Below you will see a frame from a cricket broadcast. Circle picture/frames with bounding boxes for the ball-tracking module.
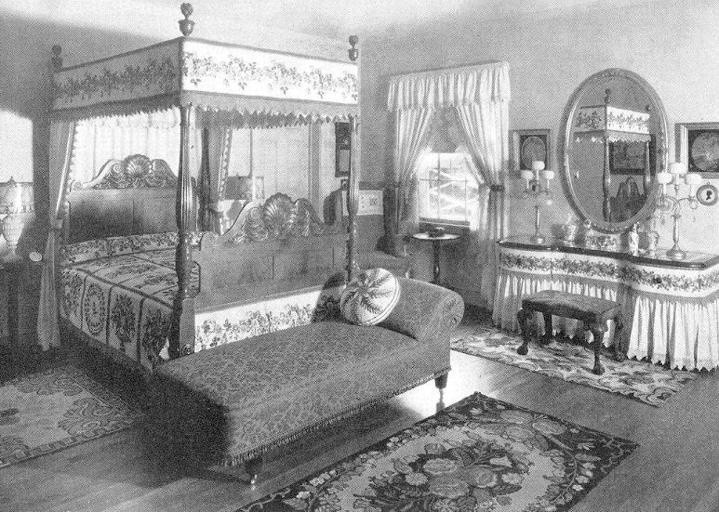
[607,133,655,178]
[677,121,719,180]
[510,127,553,175]
[696,182,719,207]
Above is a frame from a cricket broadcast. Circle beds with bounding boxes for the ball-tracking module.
[40,154,359,376]
[150,274,462,484]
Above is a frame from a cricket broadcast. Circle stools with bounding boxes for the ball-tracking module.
[518,282,624,373]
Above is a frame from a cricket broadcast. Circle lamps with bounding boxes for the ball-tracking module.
[659,163,707,261]
[521,159,555,244]
[0,177,35,264]
[228,174,265,202]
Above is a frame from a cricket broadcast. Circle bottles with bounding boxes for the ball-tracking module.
[560,218,660,256]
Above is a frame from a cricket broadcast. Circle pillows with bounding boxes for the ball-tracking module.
[338,266,403,325]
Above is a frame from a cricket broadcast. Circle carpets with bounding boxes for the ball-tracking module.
[1,361,149,468]
[230,389,641,511]
[450,324,697,405]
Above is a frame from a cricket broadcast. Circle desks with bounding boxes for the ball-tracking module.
[497,232,719,352]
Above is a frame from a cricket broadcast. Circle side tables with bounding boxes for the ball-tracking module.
[411,231,465,291]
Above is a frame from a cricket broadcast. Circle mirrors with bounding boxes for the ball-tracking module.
[556,66,670,236]
